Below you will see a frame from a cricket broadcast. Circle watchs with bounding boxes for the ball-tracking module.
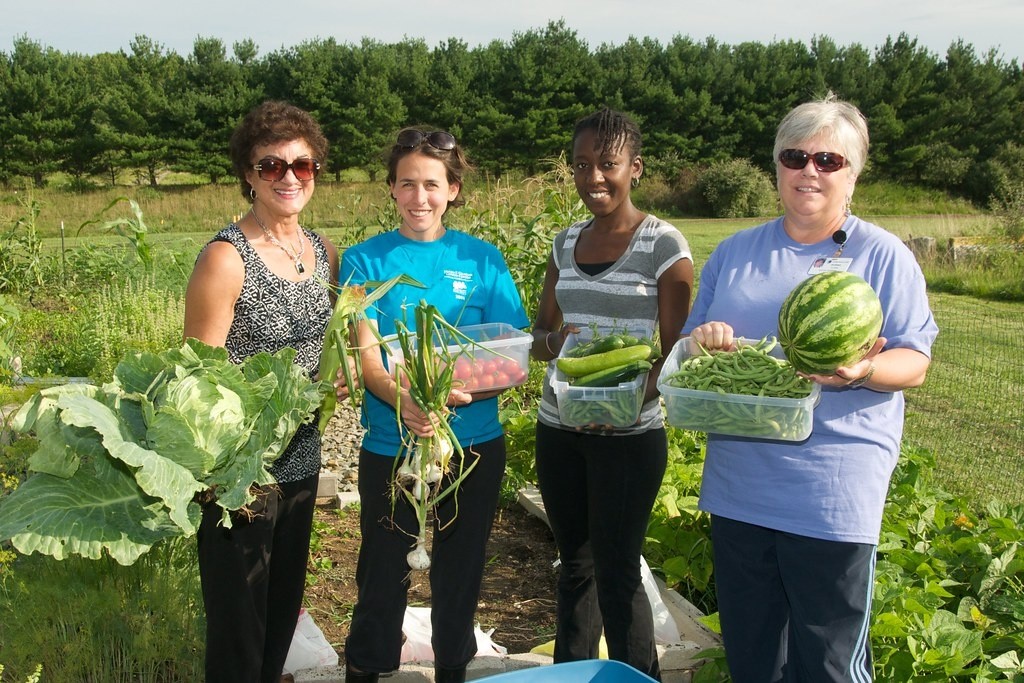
[848,366,875,389]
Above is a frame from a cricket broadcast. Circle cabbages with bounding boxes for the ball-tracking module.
[2,337,335,566]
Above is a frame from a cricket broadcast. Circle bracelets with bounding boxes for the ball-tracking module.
[546,331,561,357]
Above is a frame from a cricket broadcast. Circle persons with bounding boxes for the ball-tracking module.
[338,124,531,683]
[813,258,826,267]
[529,108,695,683]
[680,91,938,683]
[183,104,359,683]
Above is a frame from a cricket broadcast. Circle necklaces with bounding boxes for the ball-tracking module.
[250,206,305,275]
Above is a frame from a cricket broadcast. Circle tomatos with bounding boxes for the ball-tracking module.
[392,358,526,391]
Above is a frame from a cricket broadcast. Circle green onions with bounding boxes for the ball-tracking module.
[350,287,519,584]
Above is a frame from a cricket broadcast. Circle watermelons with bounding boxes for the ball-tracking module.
[779,271,884,376]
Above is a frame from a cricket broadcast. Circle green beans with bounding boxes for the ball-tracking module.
[659,333,814,440]
[567,319,630,353]
[560,388,642,426]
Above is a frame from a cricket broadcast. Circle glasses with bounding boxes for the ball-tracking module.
[778,148,852,172]
[247,156,321,182]
[395,128,457,150]
